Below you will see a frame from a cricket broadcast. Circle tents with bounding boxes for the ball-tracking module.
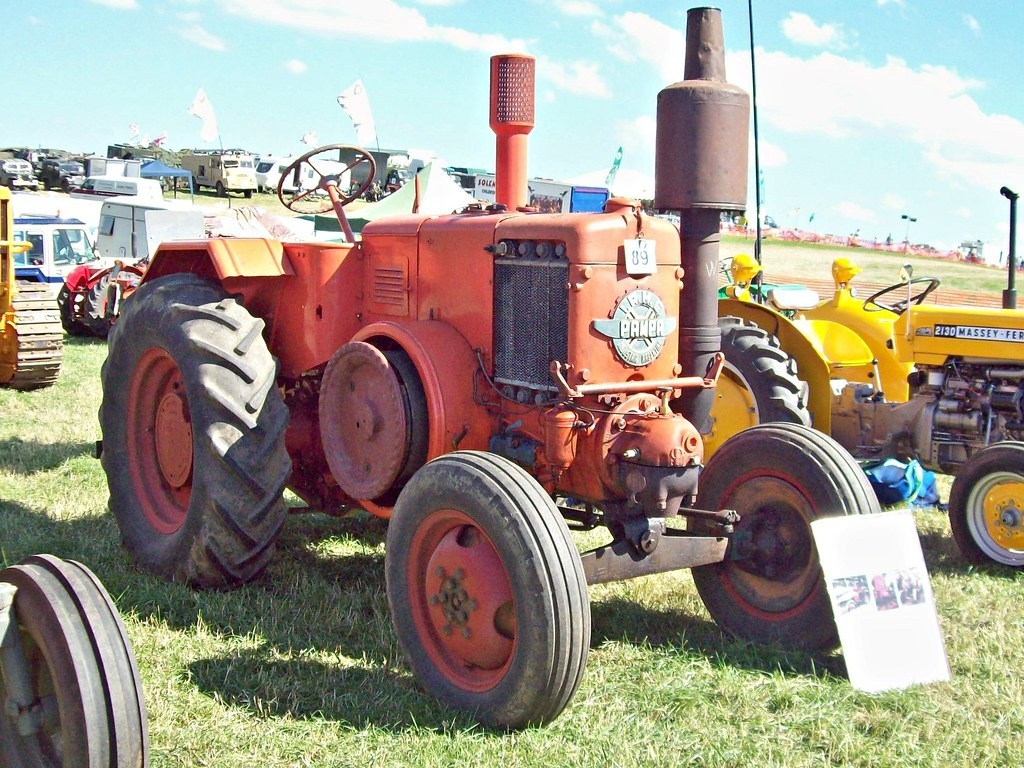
[140,160,194,203]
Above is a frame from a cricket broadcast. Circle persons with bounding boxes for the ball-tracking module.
[367,179,381,203]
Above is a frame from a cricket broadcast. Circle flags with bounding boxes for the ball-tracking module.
[302,134,321,149]
[336,80,376,149]
[183,90,218,143]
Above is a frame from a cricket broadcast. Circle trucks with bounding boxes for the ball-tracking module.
[32,156,86,193]
[180,148,259,200]
[255,156,352,198]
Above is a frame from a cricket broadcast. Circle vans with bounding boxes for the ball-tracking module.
[0,158,33,187]
[9,211,96,284]
[68,175,165,208]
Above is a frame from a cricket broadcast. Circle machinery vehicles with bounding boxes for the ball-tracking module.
[1,0,1024,768]
